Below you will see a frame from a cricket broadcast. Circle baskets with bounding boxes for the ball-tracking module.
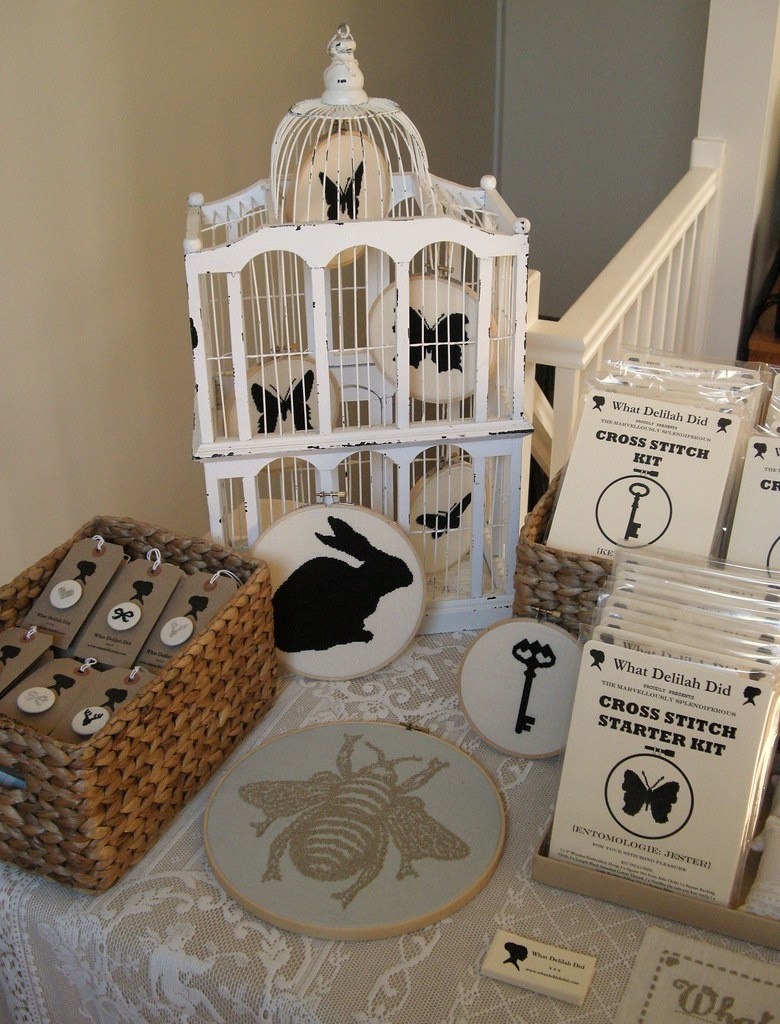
[512,469,613,639]
[0,514,281,896]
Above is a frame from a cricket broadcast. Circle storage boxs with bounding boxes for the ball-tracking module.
[533,742,780,953]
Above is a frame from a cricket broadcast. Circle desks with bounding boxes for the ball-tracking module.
[0,634,780,1024]
